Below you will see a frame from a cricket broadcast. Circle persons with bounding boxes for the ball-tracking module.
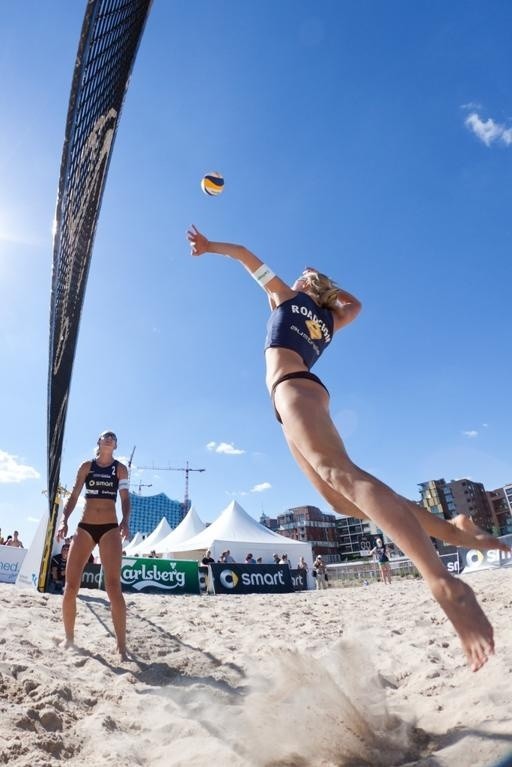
[51,426,134,664]
[182,219,512,674]
[368,538,393,587]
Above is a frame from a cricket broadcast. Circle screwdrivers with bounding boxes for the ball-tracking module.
[200,172,224,196]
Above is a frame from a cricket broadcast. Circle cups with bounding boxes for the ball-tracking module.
[103,433,116,440]
